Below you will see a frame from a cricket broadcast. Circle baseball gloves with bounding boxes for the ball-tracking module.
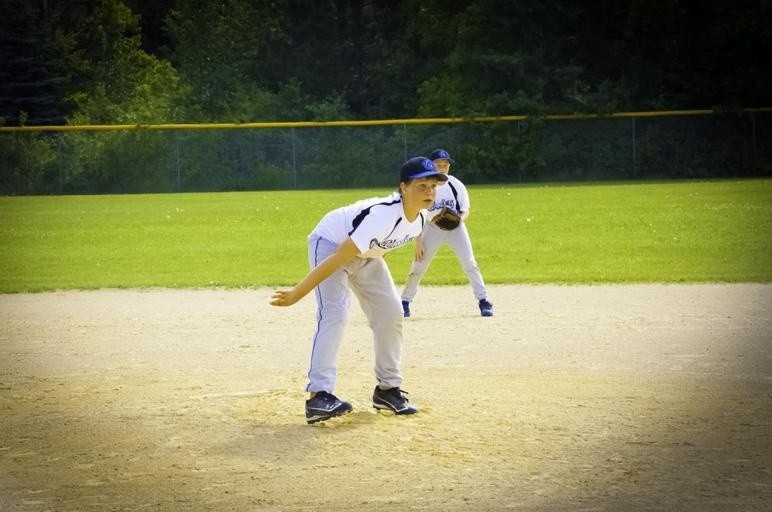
[432,208,460,230]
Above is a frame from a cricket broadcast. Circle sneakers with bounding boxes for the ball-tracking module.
[479,299,493,316]
[305,390,353,425]
[402,300,410,317]
[372,384,417,415]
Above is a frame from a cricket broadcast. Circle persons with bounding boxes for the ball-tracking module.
[271,156,449,424]
[400,149,494,318]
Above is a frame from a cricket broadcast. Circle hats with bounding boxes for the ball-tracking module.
[399,149,455,180]
